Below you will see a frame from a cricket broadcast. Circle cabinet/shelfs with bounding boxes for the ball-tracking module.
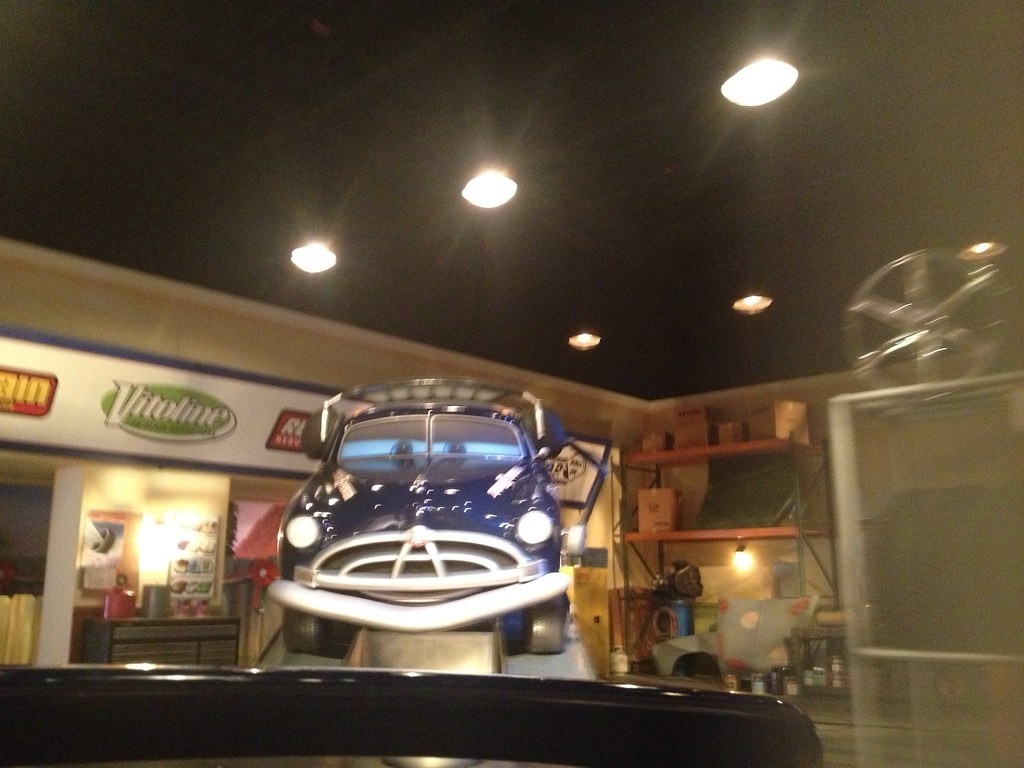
[618,428,841,686]
[79,616,241,665]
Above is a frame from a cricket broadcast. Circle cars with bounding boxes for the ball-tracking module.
[263,375,606,655]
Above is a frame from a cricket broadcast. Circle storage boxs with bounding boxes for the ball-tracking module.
[637,487,685,533]
[748,399,810,446]
[641,430,671,455]
[671,404,718,450]
[716,421,748,444]
